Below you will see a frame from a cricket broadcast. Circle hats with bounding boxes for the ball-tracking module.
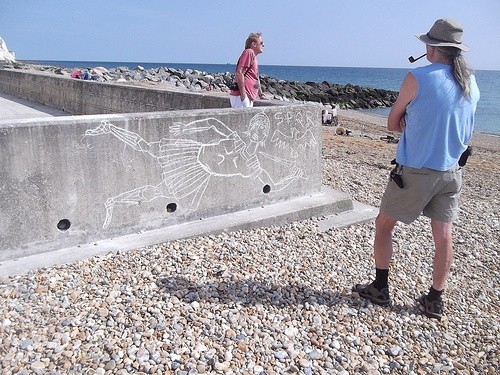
[413,19,473,52]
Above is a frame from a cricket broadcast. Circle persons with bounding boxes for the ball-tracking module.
[352,18,481,320]
[71,68,82,79]
[331,105,339,125]
[229,32,270,109]
[84,68,93,80]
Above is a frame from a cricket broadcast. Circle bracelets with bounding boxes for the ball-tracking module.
[259,93,266,98]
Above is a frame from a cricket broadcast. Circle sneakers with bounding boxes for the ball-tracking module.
[354,280,392,304]
[420,292,443,319]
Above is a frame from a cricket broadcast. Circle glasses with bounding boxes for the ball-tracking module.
[255,41,263,45]
